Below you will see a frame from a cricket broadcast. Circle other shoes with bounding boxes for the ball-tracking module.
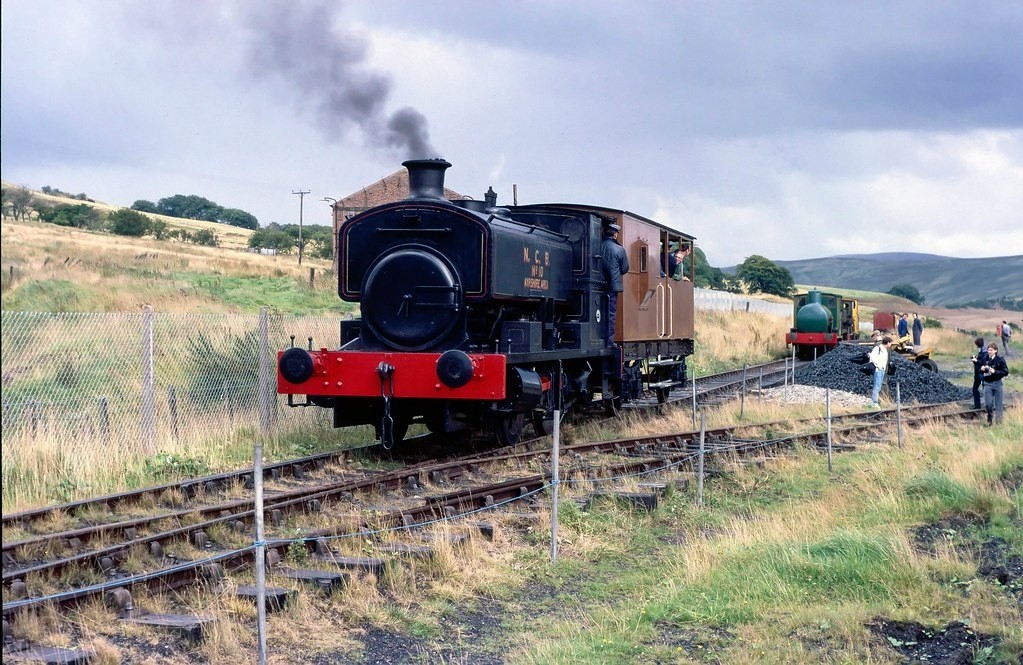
[987,419,992,426]
[873,403,880,408]
[606,342,618,348]
[970,406,981,409]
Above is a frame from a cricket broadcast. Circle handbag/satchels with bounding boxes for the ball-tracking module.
[887,361,897,375]
[860,362,876,375]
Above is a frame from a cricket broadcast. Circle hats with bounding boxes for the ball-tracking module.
[662,241,674,248]
[871,330,880,337]
[609,223,621,233]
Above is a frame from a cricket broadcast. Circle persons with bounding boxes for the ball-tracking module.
[660,241,691,282]
[870,330,883,349]
[600,224,629,347]
[971,338,1009,425]
[913,313,923,345]
[870,337,893,407]
[1002,320,1011,354]
[898,313,910,337]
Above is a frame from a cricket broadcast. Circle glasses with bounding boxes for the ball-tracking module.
[689,250,692,252]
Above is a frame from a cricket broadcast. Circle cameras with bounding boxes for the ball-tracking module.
[970,356,976,359]
[984,367,989,373]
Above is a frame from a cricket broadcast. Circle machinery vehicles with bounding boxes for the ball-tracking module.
[860,333,941,372]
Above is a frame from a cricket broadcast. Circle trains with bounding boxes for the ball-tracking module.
[786,286,860,356]
[274,158,698,451]
